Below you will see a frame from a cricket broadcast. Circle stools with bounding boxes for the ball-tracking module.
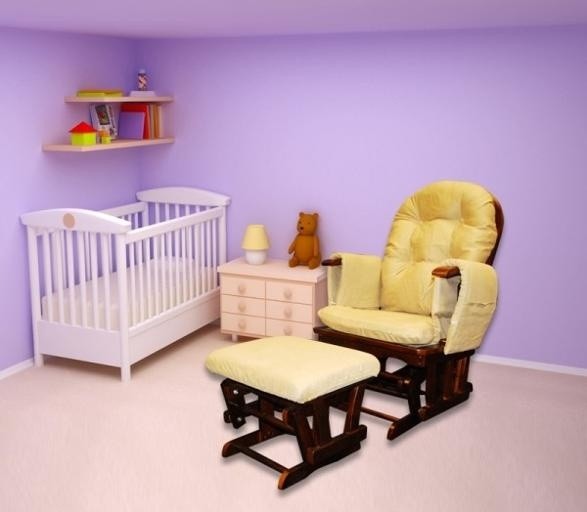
[204,337,381,486]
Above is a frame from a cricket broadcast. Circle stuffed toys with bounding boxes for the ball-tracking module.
[288,211,321,270]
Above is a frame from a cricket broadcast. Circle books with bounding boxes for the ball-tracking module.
[77,90,123,98]
[118,102,163,140]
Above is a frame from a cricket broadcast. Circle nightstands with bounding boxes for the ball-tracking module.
[215,257,325,340]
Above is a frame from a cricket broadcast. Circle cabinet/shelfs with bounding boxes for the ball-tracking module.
[40,88,174,154]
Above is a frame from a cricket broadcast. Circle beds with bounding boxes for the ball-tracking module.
[19,188,231,382]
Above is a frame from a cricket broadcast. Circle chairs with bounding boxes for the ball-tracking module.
[313,181,505,441]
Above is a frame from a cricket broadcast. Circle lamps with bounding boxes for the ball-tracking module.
[244,225,269,263]
